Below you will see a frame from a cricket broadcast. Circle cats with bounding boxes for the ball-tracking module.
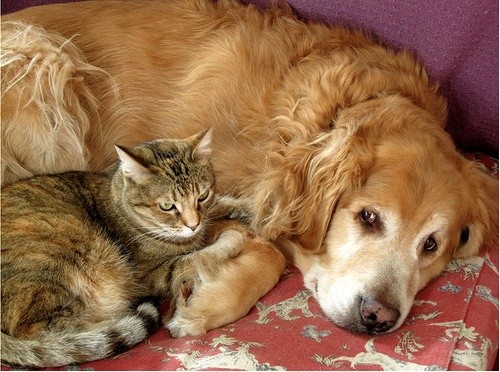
[0,125,257,371]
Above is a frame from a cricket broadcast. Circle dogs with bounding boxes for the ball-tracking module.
[1,0,499,339]
